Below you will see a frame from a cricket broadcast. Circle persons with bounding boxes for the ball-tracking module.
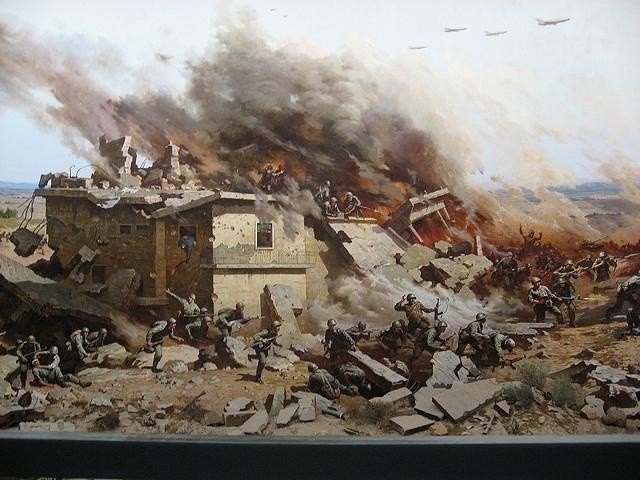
[306,294,516,400]
[255,162,286,195]
[136,288,282,386]
[313,179,362,221]
[16,326,107,389]
[491,222,640,337]
[178,236,197,265]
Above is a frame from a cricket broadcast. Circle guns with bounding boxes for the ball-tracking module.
[136,340,163,354]
[562,267,589,279]
[243,334,282,351]
[542,295,575,299]
[347,202,377,211]
[434,299,442,321]
[437,332,456,348]
[183,315,217,317]
[239,315,265,323]
[603,256,618,263]
[322,340,330,356]
[492,353,525,372]
[352,329,381,335]
[16,349,48,364]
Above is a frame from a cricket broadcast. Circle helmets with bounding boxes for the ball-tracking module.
[364,383,372,395]
[327,294,515,351]
[507,252,640,285]
[272,320,281,326]
[82,327,108,334]
[27,335,58,354]
[167,294,245,324]
[308,363,319,373]
[325,180,353,202]
